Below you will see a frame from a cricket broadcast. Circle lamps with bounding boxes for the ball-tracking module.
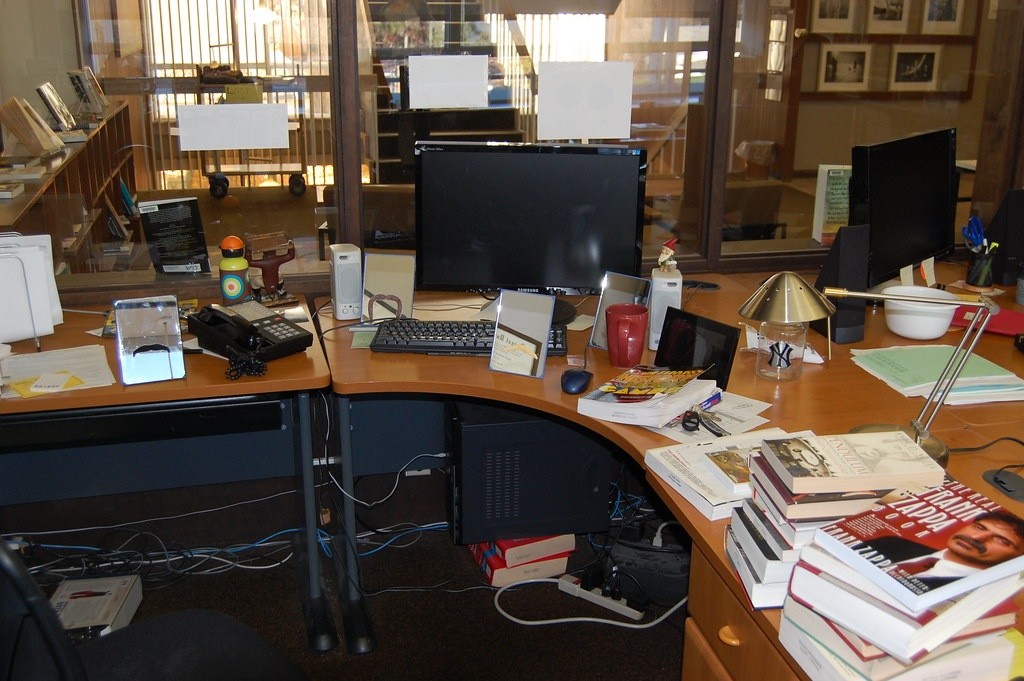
[738,270,1001,466]
[765,13,786,104]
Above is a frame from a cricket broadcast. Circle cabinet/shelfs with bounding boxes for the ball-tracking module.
[194,64,306,198]
[0,97,137,277]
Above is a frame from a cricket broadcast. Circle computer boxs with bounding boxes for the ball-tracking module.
[445,394,609,546]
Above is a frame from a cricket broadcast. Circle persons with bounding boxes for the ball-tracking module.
[849,510,1024,595]
[904,57,931,82]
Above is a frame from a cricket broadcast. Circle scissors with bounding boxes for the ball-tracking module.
[962,216,984,250]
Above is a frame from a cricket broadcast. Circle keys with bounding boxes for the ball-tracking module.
[689,405,722,422]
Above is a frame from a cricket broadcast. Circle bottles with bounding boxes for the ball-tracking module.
[219,235,251,307]
[755,320,807,380]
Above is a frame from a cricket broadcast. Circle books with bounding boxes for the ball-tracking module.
[812,165,855,247]
[644,427,1024,681]
[0,66,139,274]
[139,197,212,275]
[577,365,719,429]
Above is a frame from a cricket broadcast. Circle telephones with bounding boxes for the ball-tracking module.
[187,301,314,362]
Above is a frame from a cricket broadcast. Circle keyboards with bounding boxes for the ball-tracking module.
[368,320,567,356]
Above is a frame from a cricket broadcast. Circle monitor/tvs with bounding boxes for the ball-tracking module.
[848,127,955,304]
[413,141,647,322]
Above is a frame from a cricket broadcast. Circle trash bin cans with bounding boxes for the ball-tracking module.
[742,141,775,180]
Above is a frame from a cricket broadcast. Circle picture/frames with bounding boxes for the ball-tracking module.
[887,42,943,93]
[867,0,911,34]
[920,0,966,36]
[816,43,875,90]
[809,1,857,32]
[111,0,144,59]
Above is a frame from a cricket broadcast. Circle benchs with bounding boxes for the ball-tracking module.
[318,181,416,262]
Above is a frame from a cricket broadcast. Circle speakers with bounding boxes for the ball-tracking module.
[328,243,363,321]
[984,189,1024,286]
[807,224,871,344]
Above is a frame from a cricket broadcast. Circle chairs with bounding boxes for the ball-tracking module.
[719,179,789,241]
[1,543,284,681]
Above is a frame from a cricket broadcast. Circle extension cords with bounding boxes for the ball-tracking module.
[557,573,646,621]
[607,536,691,577]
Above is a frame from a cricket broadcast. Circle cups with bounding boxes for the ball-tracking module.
[605,303,649,368]
[1016,262,1024,305]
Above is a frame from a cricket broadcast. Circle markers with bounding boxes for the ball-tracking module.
[968,241,999,283]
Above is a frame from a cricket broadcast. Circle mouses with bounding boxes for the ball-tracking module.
[561,368,593,395]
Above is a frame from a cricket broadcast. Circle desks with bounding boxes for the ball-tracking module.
[315,271,1024,681]
[950,157,978,231]
[0,296,339,650]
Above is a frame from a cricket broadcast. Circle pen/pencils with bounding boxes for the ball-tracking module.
[983,238,989,254]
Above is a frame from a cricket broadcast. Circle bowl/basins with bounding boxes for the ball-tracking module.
[881,285,961,339]
